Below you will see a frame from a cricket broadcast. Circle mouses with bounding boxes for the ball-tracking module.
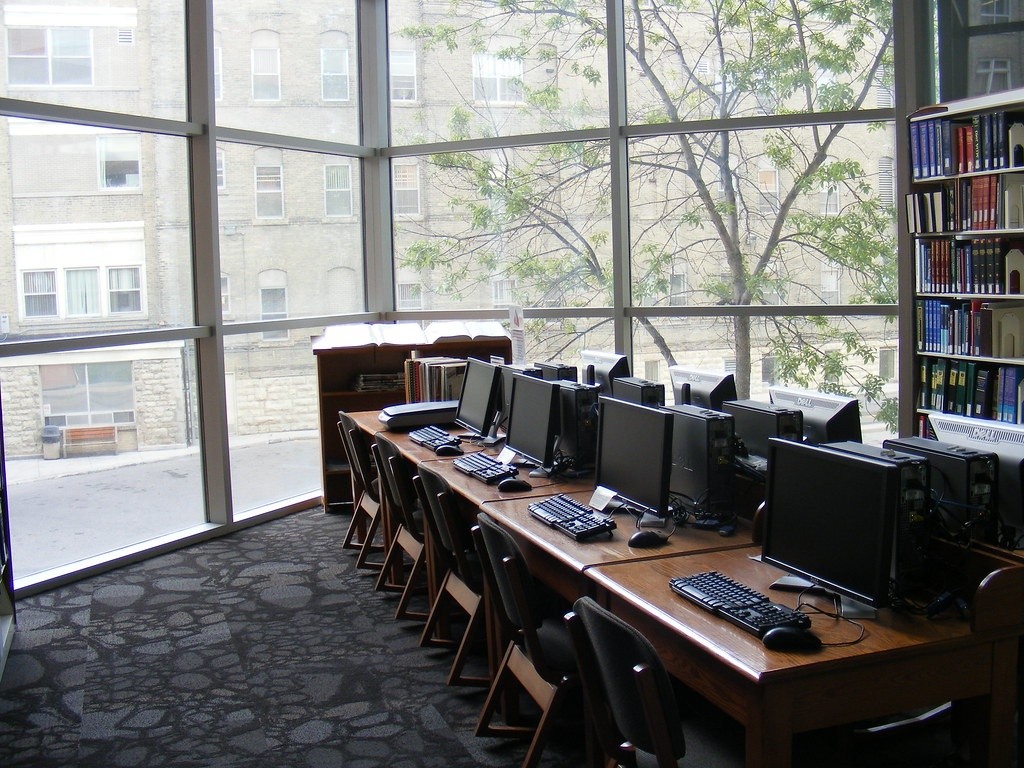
[762,626,822,650]
[435,445,464,456]
[669,570,811,637]
[628,530,668,548]
[498,479,532,490]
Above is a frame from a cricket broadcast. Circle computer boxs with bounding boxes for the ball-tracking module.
[498,361,1001,602]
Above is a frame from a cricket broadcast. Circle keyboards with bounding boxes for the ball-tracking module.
[527,493,617,541]
[452,451,519,484]
[408,426,462,451]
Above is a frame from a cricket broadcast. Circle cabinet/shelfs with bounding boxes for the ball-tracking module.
[906,86,1024,440]
[313,328,513,513]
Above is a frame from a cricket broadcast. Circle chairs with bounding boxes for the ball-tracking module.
[370,431,465,620]
[336,411,403,569]
[470,511,580,768]
[564,595,686,768]
[412,464,490,687]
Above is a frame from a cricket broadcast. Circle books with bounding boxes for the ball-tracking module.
[905,111,1024,425]
[325,356,468,506]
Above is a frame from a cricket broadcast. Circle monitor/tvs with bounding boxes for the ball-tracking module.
[761,438,897,619]
[593,396,673,527]
[769,385,863,445]
[504,372,560,478]
[669,365,737,412]
[453,356,505,446]
[581,350,631,398]
[926,412,1024,549]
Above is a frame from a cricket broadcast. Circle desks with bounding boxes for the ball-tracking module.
[343,411,1023,768]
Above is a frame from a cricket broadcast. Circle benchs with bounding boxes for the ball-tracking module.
[62,426,118,458]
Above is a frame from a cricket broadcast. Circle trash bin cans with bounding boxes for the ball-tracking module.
[41,425,61,460]
